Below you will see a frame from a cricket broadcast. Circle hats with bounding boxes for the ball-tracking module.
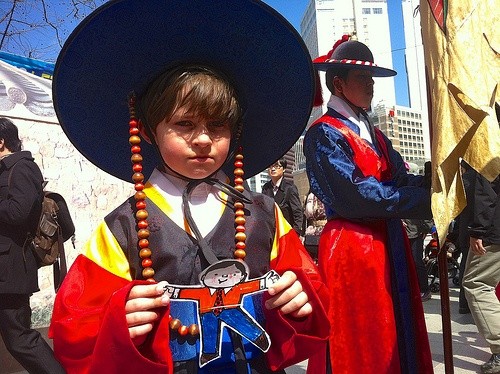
[50,0,315,188]
[312,31,397,77]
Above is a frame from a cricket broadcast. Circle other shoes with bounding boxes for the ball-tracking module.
[420,290,432,302]
[481,354,500,374]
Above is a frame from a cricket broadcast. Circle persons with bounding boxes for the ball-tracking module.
[303,31,434,374]
[401,160,500,374]
[48,0,333,374]
[262,157,303,238]
[0,118,75,374]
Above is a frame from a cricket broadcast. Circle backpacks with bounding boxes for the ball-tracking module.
[31,190,75,266]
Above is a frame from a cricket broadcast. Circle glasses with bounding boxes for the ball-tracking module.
[268,164,283,169]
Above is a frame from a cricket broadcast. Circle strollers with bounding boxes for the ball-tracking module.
[423,220,463,293]
